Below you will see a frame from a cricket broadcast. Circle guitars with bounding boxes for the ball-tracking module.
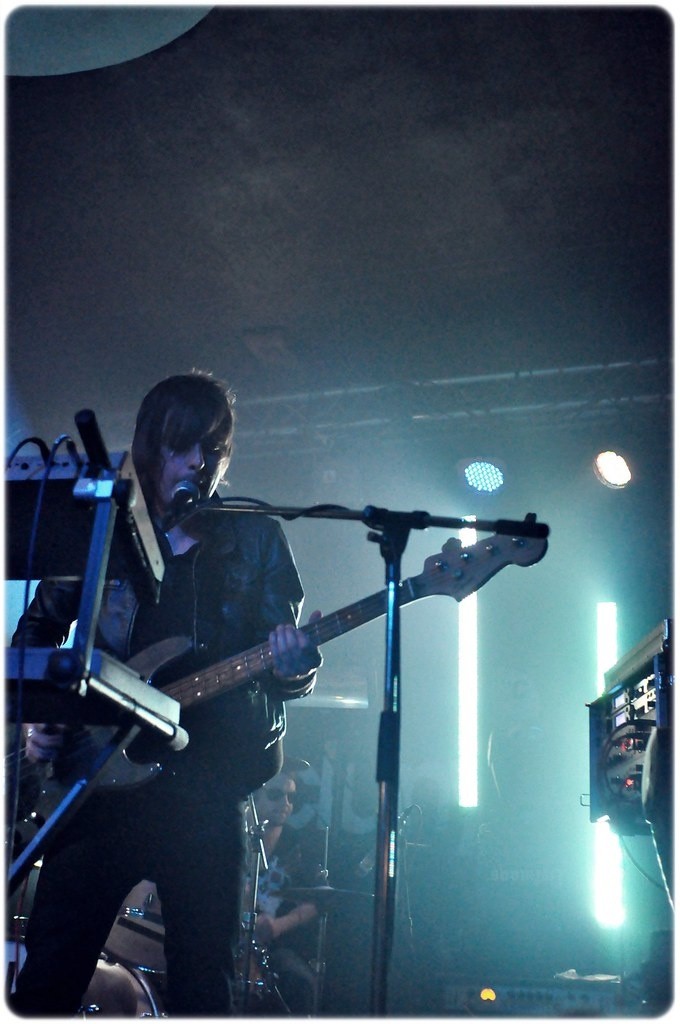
[4,513,549,864]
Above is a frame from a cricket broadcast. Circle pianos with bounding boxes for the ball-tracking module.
[5,452,164,581]
[5,643,182,731]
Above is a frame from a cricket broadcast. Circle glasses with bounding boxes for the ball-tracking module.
[262,783,296,804]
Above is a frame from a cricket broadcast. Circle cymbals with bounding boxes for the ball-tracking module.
[282,750,310,774]
[274,885,378,904]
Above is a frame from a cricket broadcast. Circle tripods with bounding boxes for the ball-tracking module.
[233,790,292,1018]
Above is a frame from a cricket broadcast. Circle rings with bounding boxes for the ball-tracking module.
[27,728,37,738]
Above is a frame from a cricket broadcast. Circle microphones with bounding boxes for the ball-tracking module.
[161,480,201,529]
[360,805,415,873]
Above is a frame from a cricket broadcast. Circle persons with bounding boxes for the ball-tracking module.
[6,375,324,1019]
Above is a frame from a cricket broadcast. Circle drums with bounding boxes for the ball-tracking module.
[230,939,295,1017]
[71,954,167,1017]
[99,880,254,973]
